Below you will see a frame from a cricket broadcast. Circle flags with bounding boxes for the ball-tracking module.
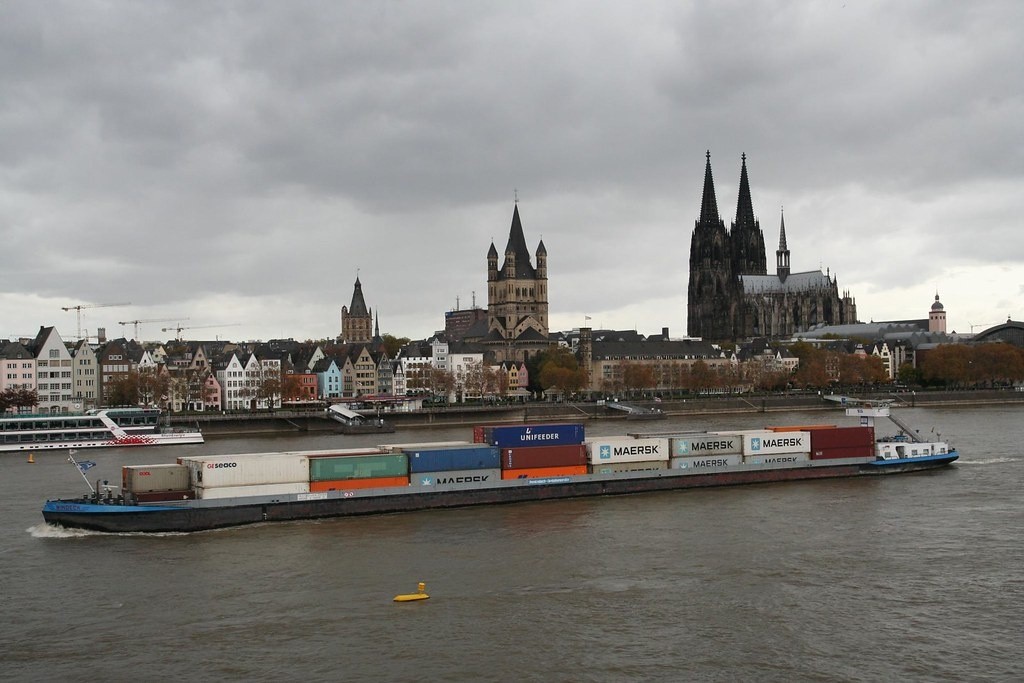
[78,460,96,473]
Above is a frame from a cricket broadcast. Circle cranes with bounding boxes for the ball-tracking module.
[10,329,98,342]
[162,322,241,341]
[61,302,132,341]
[118,317,190,341]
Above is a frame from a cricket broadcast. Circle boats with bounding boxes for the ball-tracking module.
[845,400,890,417]
[626,410,668,421]
[40,398,959,529]
[343,419,395,434]
[0,408,206,452]
